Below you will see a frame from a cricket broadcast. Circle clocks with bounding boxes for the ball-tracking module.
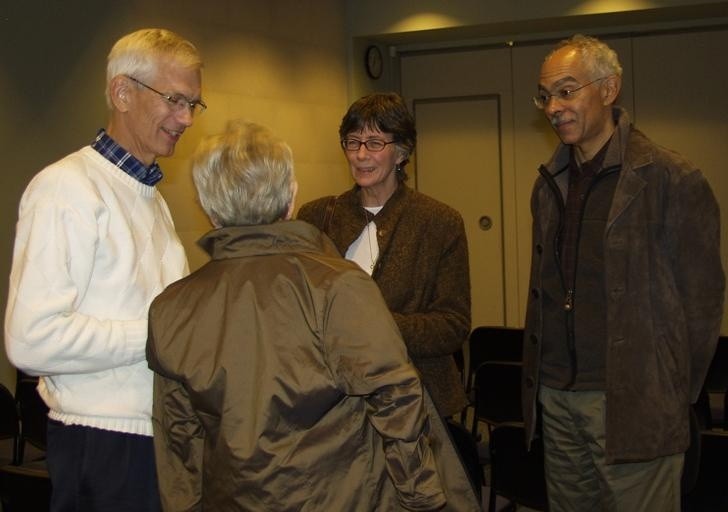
[367,46,383,80]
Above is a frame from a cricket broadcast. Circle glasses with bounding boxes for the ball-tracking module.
[532,76,606,110]
[340,139,398,151]
[126,74,206,115]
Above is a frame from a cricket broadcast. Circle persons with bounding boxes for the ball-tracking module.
[3,27,207,512]
[519,32,722,512]
[141,116,479,512]
[295,90,474,439]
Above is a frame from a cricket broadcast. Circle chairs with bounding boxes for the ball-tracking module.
[464,326,526,401]
[469,358,528,438]
[485,424,556,511]
[0,368,53,512]
[688,429,726,510]
[694,334,727,421]
